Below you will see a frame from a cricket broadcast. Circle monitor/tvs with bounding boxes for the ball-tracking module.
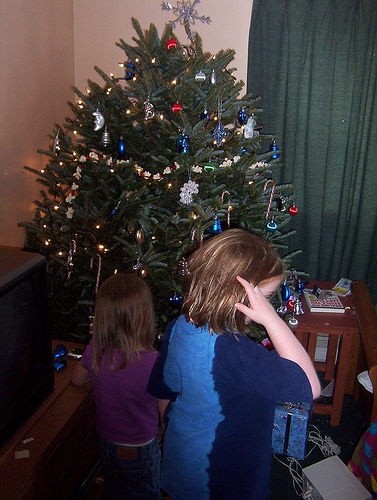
[0,246,56,450]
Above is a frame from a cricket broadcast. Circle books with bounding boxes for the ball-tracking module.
[303,289,345,314]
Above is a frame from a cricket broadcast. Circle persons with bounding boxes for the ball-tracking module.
[146,228,322,500]
[72,274,164,500]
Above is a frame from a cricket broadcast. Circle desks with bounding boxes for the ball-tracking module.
[285,280,360,427]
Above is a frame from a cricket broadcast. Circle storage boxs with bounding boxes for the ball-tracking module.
[271,403,312,459]
[301,456,372,500]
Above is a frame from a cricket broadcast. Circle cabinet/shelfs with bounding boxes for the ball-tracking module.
[0,340,103,500]
